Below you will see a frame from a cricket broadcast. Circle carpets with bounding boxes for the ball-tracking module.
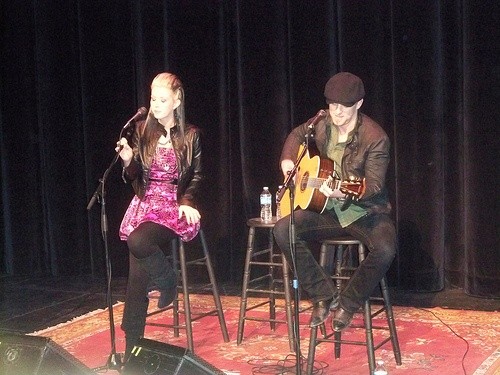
[26,289,500,375]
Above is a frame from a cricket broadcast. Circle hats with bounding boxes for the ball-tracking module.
[323,72,366,107]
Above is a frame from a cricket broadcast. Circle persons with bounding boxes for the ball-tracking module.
[115,72,205,366]
[274,72,396,332]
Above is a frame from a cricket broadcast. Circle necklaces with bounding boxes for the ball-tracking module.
[156,139,170,145]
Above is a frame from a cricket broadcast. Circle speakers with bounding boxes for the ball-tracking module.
[0,330,99,375]
[121,335,230,375]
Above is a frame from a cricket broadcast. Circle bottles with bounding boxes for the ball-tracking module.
[374,361,388,375]
[276,185,284,221]
[259,187,272,223]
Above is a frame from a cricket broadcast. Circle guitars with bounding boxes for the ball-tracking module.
[276,142,366,220]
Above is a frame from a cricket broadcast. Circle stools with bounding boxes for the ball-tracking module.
[307,234,402,375]
[145,226,229,354]
[239,219,326,353]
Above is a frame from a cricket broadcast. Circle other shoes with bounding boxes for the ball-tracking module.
[121,335,145,364]
[157,288,177,309]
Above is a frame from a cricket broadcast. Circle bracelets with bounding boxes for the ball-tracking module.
[344,193,348,198]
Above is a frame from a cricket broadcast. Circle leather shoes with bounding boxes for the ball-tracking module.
[331,303,354,333]
[308,291,340,327]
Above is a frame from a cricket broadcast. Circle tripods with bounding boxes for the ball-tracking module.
[88,130,131,375]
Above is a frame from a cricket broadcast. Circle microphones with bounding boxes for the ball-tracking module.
[124,106,148,129]
[309,109,328,130]
